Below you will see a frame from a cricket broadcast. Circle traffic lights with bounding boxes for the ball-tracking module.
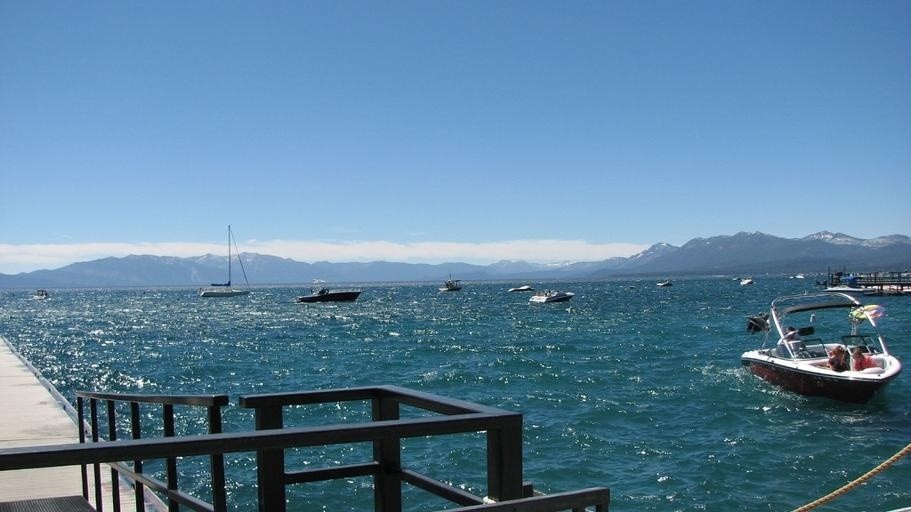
[509,282,536,293]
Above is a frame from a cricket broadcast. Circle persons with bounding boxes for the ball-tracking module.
[827,345,850,372]
[852,347,879,371]
[784,327,806,349]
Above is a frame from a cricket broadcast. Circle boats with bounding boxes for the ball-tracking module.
[823,285,880,296]
[436,273,463,292]
[32,289,48,301]
[740,290,903,405]
[295,276,362,302]
[731,274,754,286]
[528,288,577,304]
[788,272,804,279]
[656,280,672,288]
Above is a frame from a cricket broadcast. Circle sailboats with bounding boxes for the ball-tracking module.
[197,222,251,296]
